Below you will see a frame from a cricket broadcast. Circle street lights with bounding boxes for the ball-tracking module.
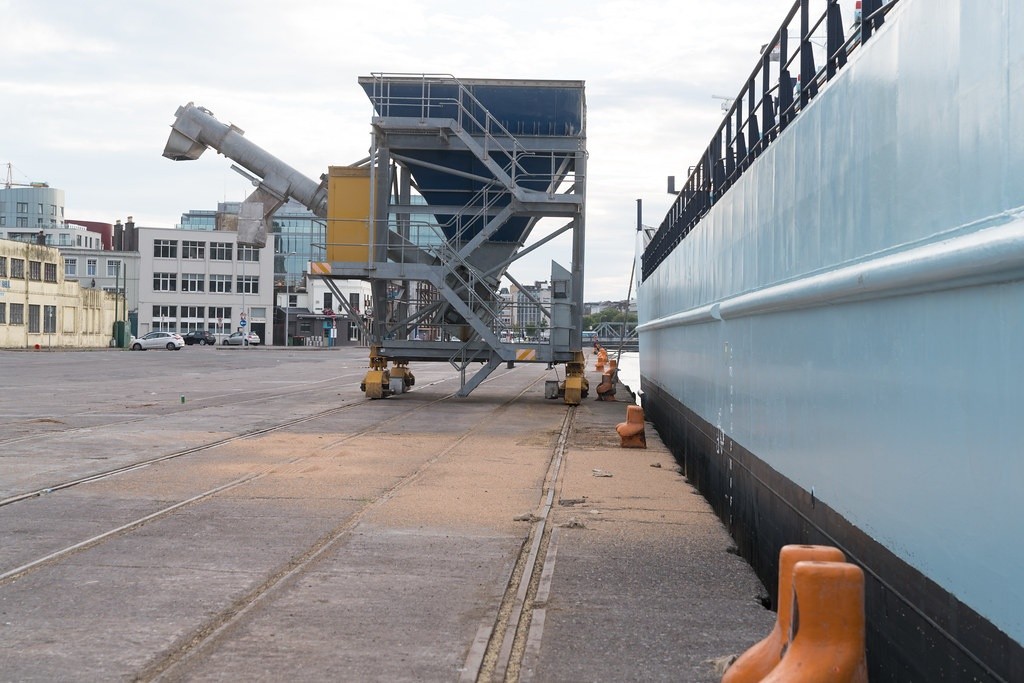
[284,251,297,347]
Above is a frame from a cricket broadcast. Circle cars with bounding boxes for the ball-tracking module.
[128,330,186,351]
[222,331,261,346]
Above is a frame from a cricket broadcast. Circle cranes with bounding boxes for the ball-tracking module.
[1,161,51,188]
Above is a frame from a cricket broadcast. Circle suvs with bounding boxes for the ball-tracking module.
[181,329,217,346]
